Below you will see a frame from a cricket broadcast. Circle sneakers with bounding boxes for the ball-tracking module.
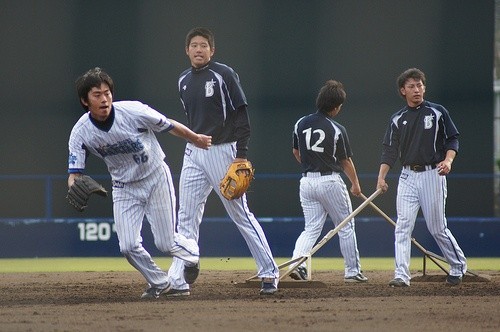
[445,275,461,285]
[142,283,170,299]
[290,268,308,280]
[166,287,190,296]
[260,276,279,295]
[183,263,200,284]
[389,278,405,286]
[344,274,369,283]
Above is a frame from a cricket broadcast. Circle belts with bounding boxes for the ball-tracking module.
[303,171,332,177]
[403,163,437,173]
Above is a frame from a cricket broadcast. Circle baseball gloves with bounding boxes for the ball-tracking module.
[220,160,255,200]
[65,175,109,213]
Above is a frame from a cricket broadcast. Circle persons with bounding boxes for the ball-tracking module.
[375,68,467,288]
[68,67,212,299]
[163,26,279,298]
[289,80,369,282]
